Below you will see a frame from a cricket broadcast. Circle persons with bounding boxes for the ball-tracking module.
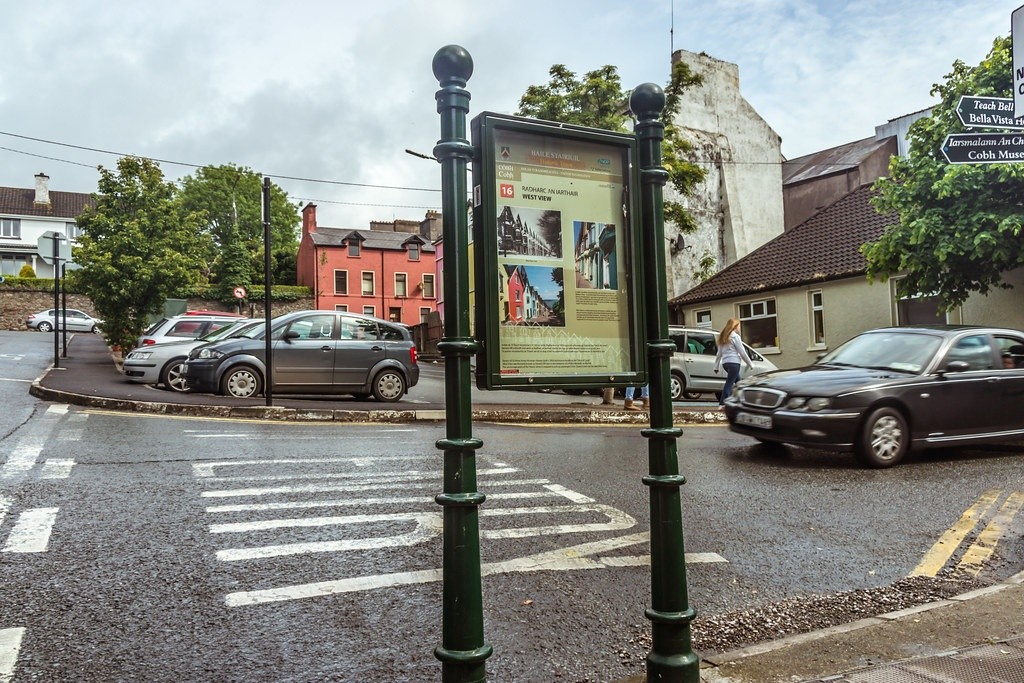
[624,384,650,411]
[714,318,754,411]
[1002,354,1016,369]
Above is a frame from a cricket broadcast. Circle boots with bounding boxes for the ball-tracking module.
[641,397,650,411]
[623,399,641,411]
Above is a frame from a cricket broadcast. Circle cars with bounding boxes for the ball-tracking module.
[121,309,354,394]
[182,308,419,403]
[723,322,1024,469]
[620,323,780,402]
[27,308,106,335]
